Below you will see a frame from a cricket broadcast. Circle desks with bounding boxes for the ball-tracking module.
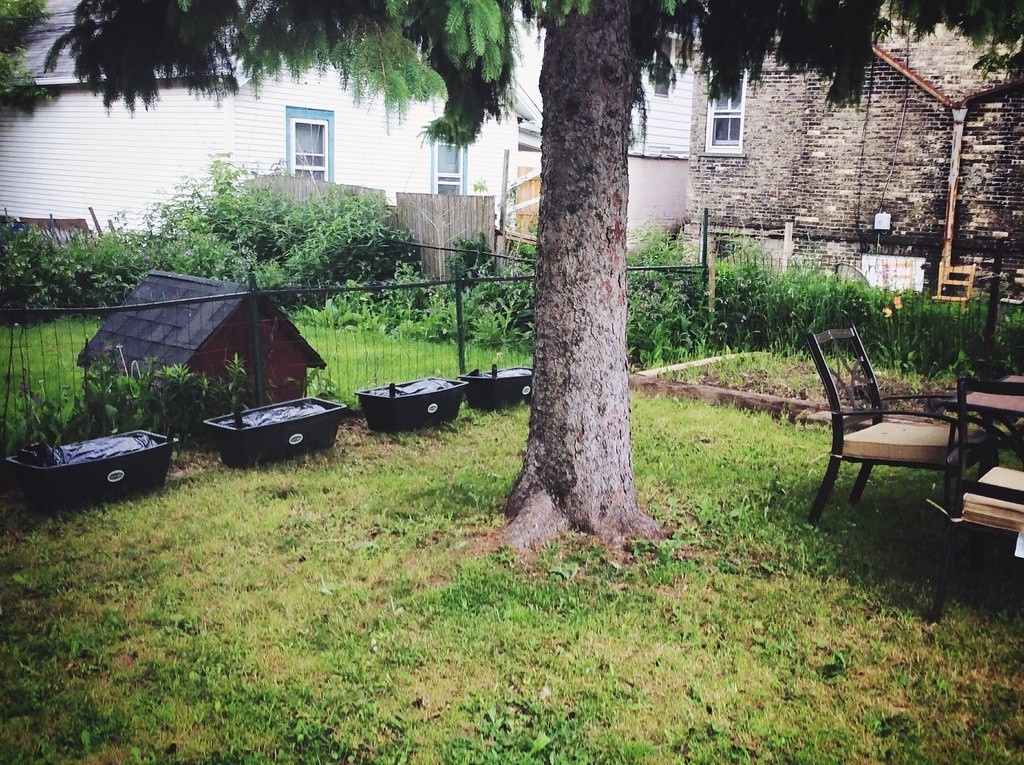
[943,372,1024,416]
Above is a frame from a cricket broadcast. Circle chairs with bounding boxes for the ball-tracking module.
[927,377,1024,624]
[805,324,999,524]
[931,261,976,303]
